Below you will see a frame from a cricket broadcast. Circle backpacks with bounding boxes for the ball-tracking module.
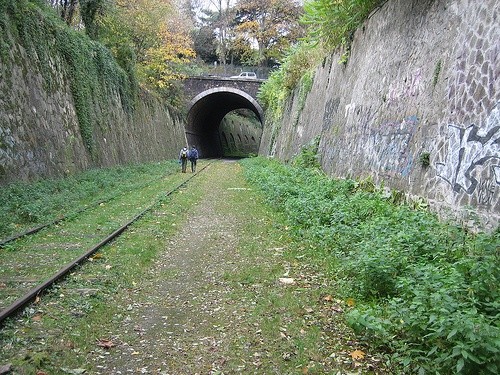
[181,149,188,158]
[190,149,196,159]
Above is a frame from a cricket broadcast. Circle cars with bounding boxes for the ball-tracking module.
[231,72,258,80]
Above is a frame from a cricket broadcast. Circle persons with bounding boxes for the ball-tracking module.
[187,144,199,172]
[179,146,190,173]
